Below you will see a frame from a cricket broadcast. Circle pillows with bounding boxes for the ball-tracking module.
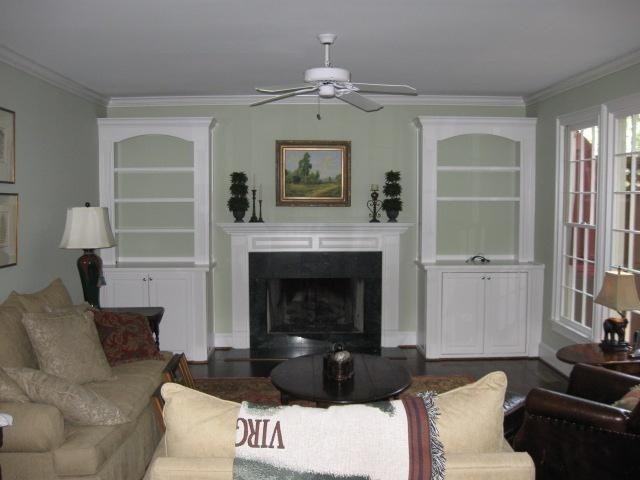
[88,309,166,367]
[2,366,133,426]
[21,311,120,385]
[45,301,95,315]
[160,370,508,458]
[610,383,640,411]
[17,277,73,313]
[0,367,31,403]
[0,289,40,369]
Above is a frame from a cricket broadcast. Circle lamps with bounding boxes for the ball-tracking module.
[593,265,640,318]
[59,201,117,307]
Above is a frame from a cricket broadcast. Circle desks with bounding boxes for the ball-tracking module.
[100,306,165,349]
[556,342,640,367]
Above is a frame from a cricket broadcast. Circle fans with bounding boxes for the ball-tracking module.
[249,32,418,121]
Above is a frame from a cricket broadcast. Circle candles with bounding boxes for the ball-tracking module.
[370,183,379,192]
[258,184,263,201]
[252,173,257,191]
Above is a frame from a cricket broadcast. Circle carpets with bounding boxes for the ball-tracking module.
[180,373,480,409]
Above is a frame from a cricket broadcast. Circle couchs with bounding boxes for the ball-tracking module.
[512,362,640,480]
[142,451,537,480]
[0,349,174,480]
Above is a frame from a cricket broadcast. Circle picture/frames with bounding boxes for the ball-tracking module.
[151,382,165,428]
[0,192,18,269]
[0,106,17,185]
[275,139,352,208]
[161,352,196,389]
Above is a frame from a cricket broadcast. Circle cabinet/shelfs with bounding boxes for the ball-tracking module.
[100,263,216,364]
[416,260,546,361]
